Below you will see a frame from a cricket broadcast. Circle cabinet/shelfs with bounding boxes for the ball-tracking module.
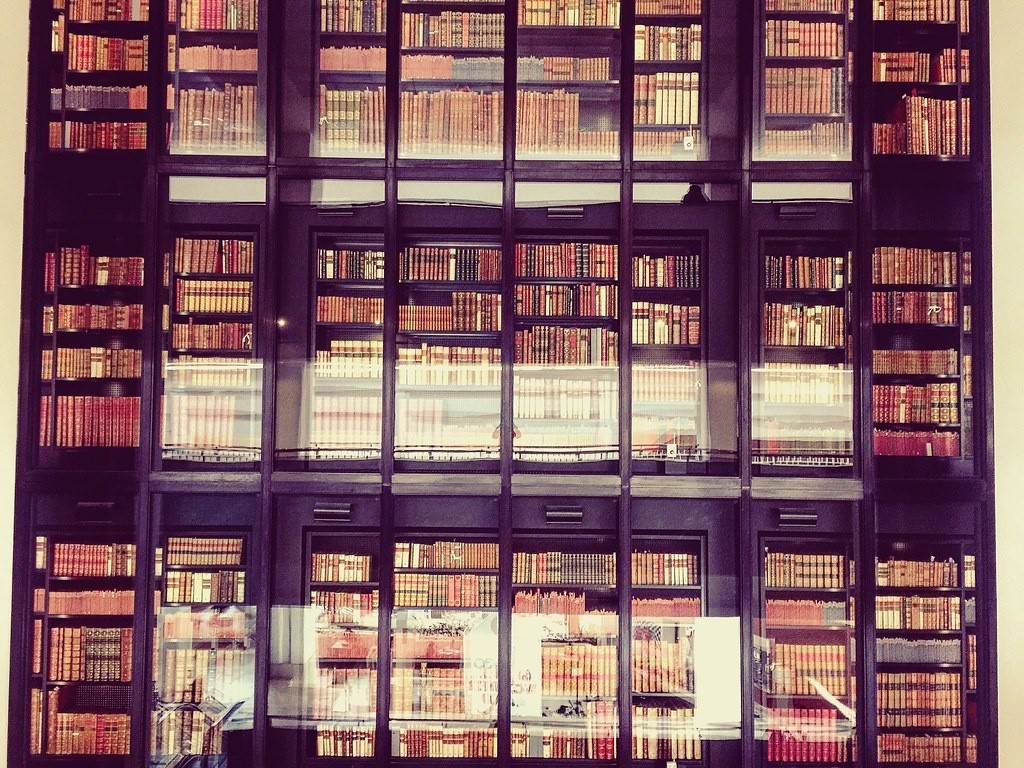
[1,0,998,768]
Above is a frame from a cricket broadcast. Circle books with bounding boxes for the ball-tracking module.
[31,1,993,763]
